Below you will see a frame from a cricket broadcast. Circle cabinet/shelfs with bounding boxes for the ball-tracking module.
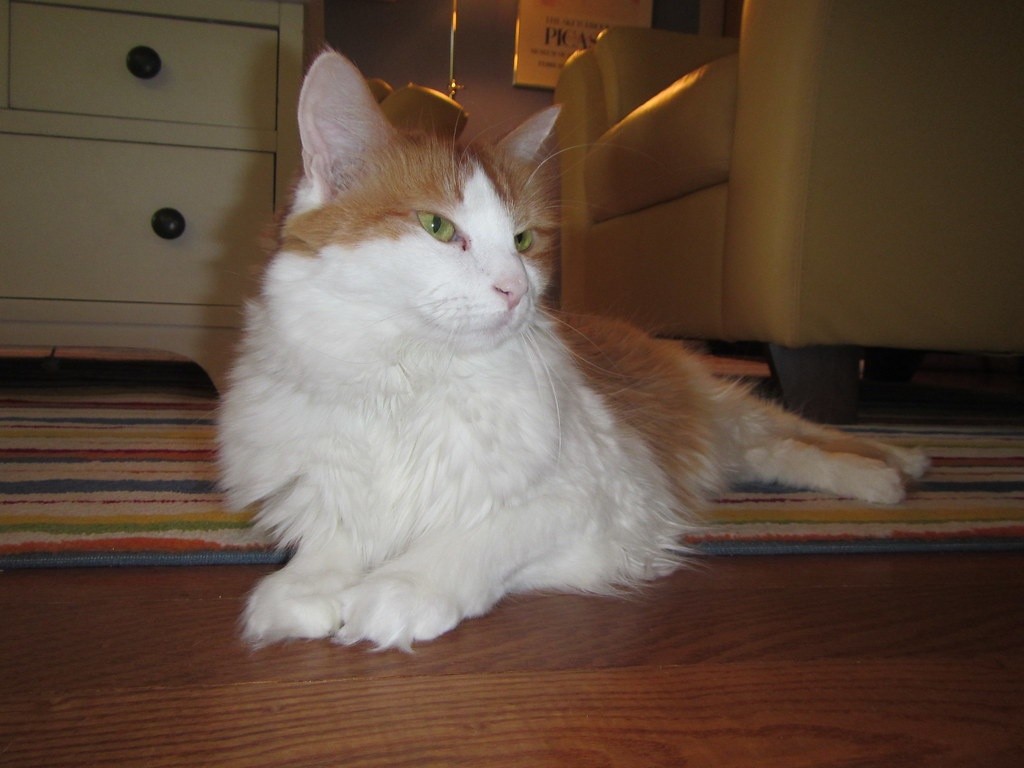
[0,0,304,397]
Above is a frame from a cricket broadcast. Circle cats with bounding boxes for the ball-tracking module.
[212,42,931,657]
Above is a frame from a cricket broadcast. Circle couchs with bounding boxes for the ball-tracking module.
[553,0,1024,425]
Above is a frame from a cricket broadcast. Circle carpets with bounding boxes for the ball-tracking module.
[0,352,1024,567]
